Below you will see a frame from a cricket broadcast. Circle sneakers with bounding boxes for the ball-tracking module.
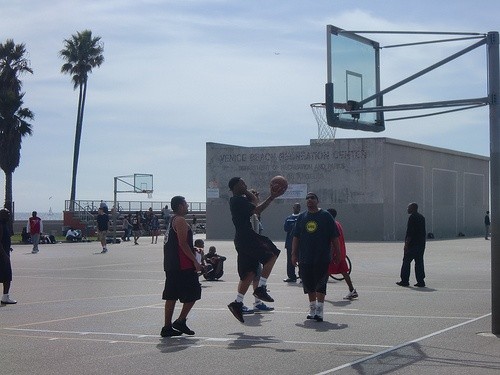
[171,317,195,335]
[227,300,245,324]
[160,325,184,338]
[252,285,275,302]
[241,305,255,314]
[253,302,275,311]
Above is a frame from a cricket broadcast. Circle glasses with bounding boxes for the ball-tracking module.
[305,196,313,200]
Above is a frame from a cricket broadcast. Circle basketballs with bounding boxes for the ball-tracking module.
[270,175,289,194]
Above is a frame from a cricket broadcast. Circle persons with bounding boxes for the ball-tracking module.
[26,211,43,253]
[122,205,170,245]
[65,227,82,242]
[100,200,109,215]
[485,211,491,240]
[96,208,109,254]
[160,196,204,337]
[283,203,304,283]
[0,208,17,304]
[192,214,197,229]
[21,227,56,244]
[193,239,227,281]
[326,208,358,299]
[227,176,281,323]
[395,202,426,287]
[289,192,341,323]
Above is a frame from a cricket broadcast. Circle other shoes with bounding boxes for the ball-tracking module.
[100,248,108,253]
[413,280,425,287]
[307,309,315,319]
[343,289,358,300]
[1,294,18,304]
[396,281,409,287]
[32,249,40,254]
[313,313,323,321]
[282,277,297,282]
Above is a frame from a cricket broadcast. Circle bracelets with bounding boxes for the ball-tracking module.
[268,196,274,201]
